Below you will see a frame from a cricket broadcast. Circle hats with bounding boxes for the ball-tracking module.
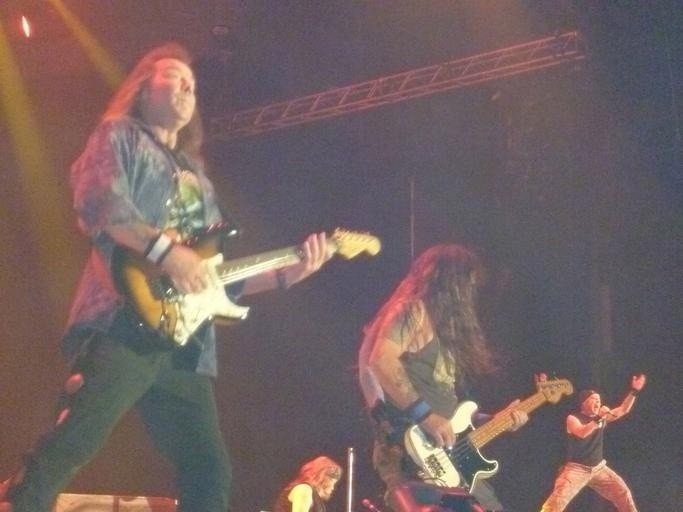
[578,389,599,405]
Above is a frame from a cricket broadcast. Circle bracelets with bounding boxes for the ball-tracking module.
[273,268,290,290]
[405,396,434,424]
[142,231,174,266]
[630,386,639,396]
[371,398,389,424]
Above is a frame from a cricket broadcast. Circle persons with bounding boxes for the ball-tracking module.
[274,454,341,511]
[369,243,529,511]
[7,43,332,511]
[537,372,646,511]
[357,310,404,488]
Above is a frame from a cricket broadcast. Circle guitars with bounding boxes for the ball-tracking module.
[110,224,381,354]
[403,375,572,494]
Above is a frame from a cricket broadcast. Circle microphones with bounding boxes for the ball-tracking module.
[600,404,617,417]
